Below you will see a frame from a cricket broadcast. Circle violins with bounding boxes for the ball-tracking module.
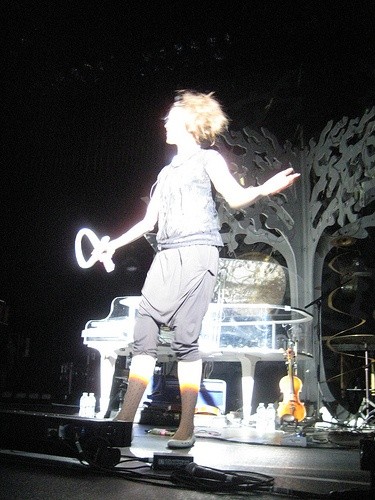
[275,346,306,423]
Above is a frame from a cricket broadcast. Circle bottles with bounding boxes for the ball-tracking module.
[79,392,89,417]
[86,393,96,418]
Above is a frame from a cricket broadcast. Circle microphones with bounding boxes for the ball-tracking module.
[185,462,237,483]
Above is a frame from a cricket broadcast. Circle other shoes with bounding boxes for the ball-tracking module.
[168,433,195,448]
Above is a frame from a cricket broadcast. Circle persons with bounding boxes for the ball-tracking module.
[101,88,302,447]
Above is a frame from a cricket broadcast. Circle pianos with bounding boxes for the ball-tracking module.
[81,294,322,432]
